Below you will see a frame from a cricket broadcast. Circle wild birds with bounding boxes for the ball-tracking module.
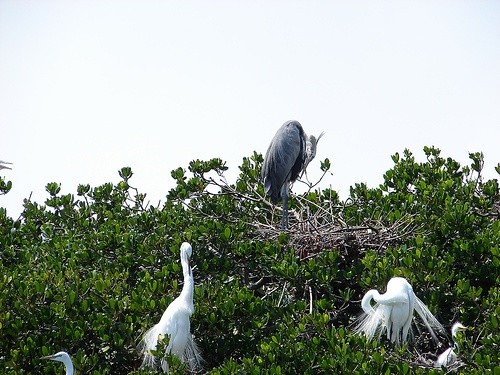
[349,277,450,351]
[437,322,468,367]
[39,351,74,375]
[134,242,207,375]
[261,120,325,228]
